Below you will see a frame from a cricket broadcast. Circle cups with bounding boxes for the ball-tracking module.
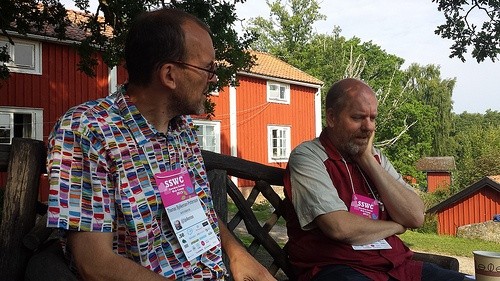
[473,251,500,281]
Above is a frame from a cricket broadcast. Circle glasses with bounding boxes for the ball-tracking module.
[156,59,217,80]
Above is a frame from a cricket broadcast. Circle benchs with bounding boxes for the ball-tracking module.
[0,138,459,281]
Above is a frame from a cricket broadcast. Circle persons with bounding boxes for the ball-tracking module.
[50,9,279,281]
[286,79,476,281]
